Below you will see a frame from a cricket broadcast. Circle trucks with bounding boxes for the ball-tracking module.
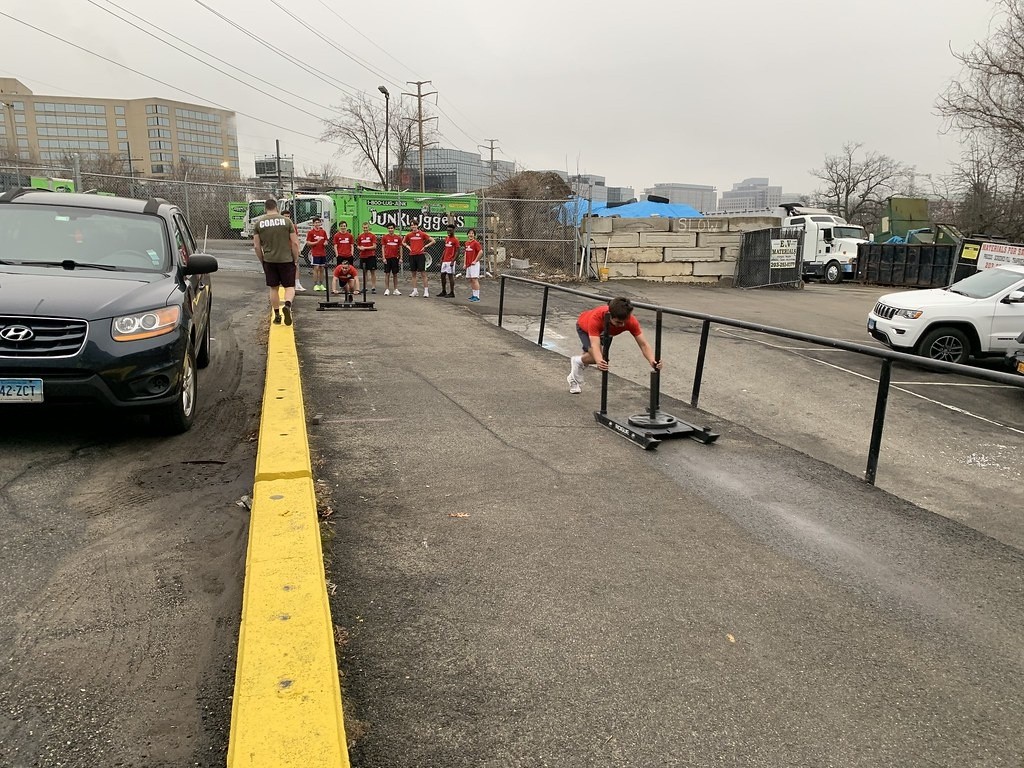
[242,200,266,238]
[285,182,480,272]
[786,215,872,285]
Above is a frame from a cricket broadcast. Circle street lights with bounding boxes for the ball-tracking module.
[377,86,390,192]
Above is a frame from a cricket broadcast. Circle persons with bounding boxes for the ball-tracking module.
[332,260,360,294]
[357,222,377,294]
[566,297,662,394]
[333,221,354,266]
[402,220,436,297]
[381,223,403,296]
[307,218,328,292]
[463,229,483,302]
[254,199,298,327]
[281,210,306,290]
[436,224,460,298]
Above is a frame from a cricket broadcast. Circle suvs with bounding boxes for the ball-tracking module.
[0,186,219,436]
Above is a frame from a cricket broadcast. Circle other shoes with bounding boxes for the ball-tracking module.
[340,285,346,294]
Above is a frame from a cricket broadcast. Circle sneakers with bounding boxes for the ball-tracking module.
[436,290,447,297]
[571,355,585,385]
[383,288,390,296]
[372,288,376,294]
[314,285,321,291]
[468,295,480,302]
[283,306,292,326]
[393,289,401,295]
[273,313,281,324]
[423,291,429,298]
[445,292,454,297]
[320,284,328,291]
[409,291,419,297]
[567,373,581,394]
[361,288,369,293]
[295,283,307,291]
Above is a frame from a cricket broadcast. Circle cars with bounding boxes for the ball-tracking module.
[866,265,1024,373]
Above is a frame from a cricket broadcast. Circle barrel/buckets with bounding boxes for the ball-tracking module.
[599,268,609,282]
